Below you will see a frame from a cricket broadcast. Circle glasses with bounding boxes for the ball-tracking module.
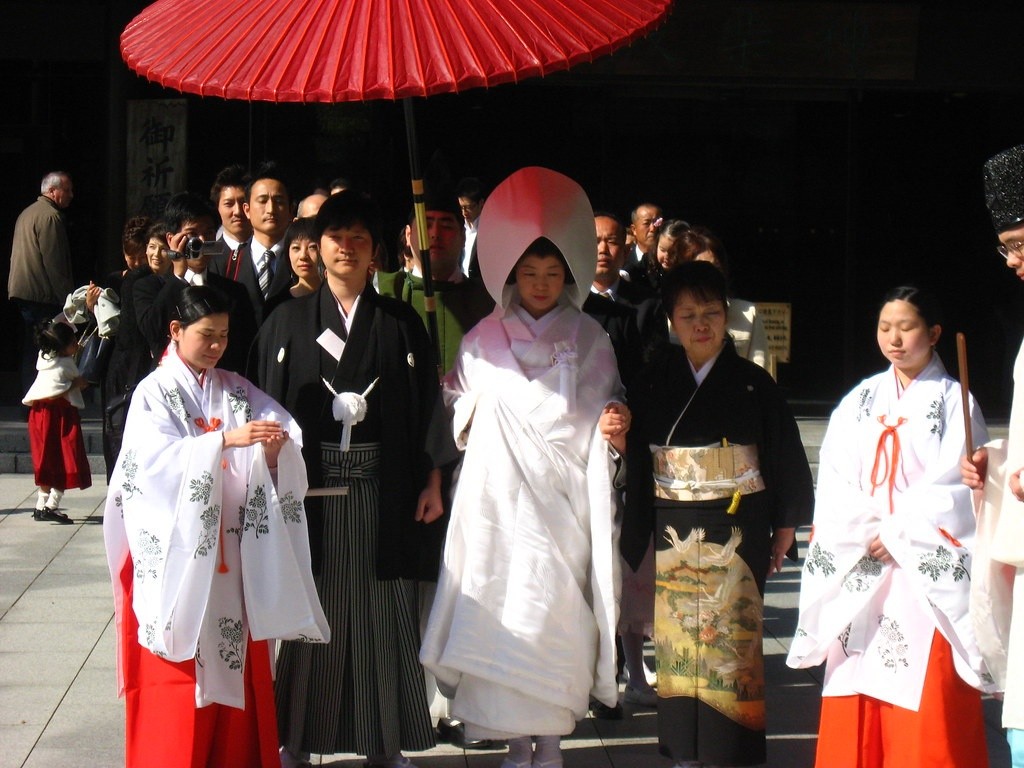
[461,203,478,212]
[997,236,1024,259]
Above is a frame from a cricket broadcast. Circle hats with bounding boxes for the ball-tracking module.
[983,145,1024,231]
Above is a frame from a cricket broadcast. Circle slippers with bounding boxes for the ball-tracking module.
[502,757,533,768]
[362,757,410,768]
[280,748,313,768]
[532,757,564,768]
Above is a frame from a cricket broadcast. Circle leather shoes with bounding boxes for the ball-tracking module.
[436,719,492,749]
[40,506,74,523]
[31,508,69,521]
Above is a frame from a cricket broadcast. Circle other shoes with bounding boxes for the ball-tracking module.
[624,681,656,705]
[622,664,658,685]
[588,693,623,719]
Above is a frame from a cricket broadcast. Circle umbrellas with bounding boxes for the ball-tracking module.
[120,0,671,383]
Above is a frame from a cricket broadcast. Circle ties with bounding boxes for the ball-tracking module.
[257,250,276,301]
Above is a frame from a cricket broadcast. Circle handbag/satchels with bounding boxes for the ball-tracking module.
[73,334,112,388]
[104,390,130,466]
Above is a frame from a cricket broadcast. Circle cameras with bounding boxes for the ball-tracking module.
[183,238,224,260]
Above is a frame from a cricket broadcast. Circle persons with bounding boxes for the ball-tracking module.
[86,162,814,768]
[785,287,990,768]
[962,145,1024,768]
[22,322,93,524]
[8,171,76,384]
